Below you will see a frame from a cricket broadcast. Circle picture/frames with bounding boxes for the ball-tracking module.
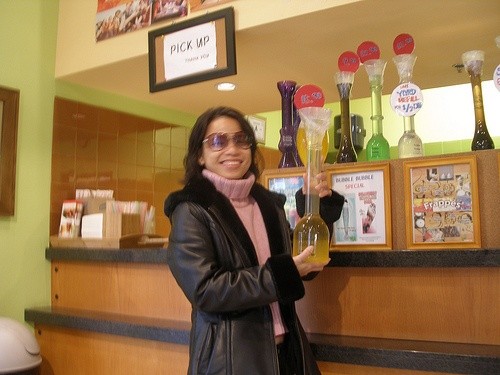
[147,6,238,94]
[259,173,308,230]
[326,163,392,252]
[0,85,20,218]
[402,152,482,252]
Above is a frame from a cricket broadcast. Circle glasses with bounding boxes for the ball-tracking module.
[202,130,254,152]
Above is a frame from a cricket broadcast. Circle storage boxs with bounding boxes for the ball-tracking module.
[79,198,141,241]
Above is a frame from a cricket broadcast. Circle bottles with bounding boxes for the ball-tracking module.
[293,84,304,166]
[391,52,424,158]
[296,118,330,165]
[334,70,359,163]
[460,49,496,152]
[276,79,296,167]
[362,57,391,161]
[292,107,335,262]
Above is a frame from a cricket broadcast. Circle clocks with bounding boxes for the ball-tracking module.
[245,114,267,145]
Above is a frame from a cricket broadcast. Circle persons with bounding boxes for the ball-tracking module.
[165,106,344,375]
[96,0,188,42]
